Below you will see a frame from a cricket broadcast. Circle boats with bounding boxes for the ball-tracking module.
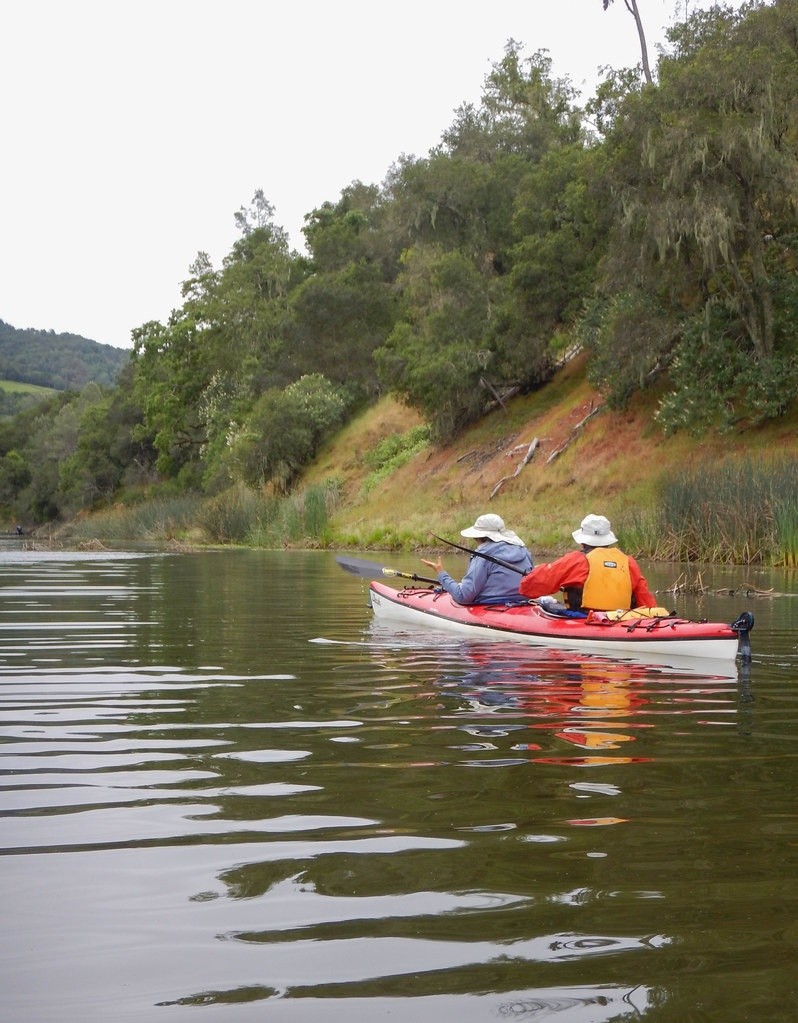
[366,581,755,665]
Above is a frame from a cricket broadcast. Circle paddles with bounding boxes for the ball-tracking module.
[428,530,567,595]
[334,552,442,586]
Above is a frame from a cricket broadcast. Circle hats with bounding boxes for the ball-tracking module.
[572,514,619,546]
[460,514,525,547]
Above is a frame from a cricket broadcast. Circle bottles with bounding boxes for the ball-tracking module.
[539,597,557,604]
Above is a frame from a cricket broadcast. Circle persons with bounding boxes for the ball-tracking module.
[420,513,534,605]
[519,513,657,614]
